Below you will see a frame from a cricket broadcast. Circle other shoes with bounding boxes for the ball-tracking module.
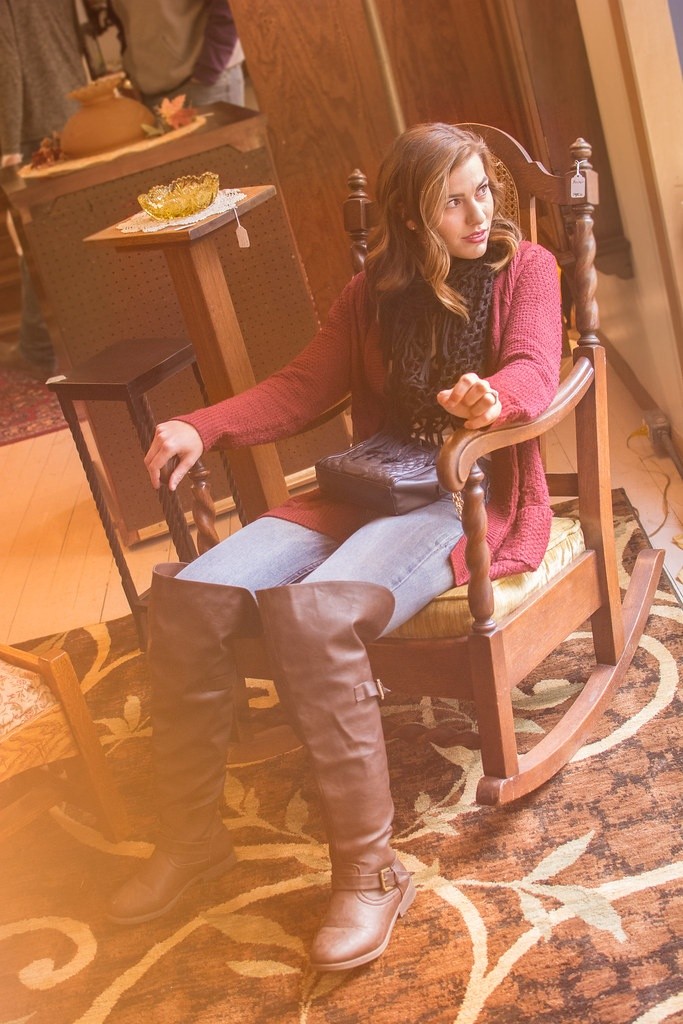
[0,340,54,377]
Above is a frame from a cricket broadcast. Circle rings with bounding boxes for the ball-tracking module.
[487,391,498,405]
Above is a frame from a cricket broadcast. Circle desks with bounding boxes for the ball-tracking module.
[86,184,293,519]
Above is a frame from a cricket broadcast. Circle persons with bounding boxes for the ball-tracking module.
[0,0,89,376]
[106,0,245,117]
[104,122,562,973]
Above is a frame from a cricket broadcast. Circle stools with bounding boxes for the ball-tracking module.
[44,337,248,651]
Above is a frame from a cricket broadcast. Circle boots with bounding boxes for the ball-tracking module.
[254,581,418,972]
[106,562,253,925]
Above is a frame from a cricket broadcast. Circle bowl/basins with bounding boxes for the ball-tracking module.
[138,173,219,222]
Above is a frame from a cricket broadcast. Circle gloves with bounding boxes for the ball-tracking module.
[171,80,211,110]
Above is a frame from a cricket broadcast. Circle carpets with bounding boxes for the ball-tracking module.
[0,484,682,1024]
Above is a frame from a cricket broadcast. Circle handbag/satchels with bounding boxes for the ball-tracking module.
[314,425,452,516]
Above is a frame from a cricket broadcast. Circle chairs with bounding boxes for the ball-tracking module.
[152,122,667,803]
[0,644,130,846]
